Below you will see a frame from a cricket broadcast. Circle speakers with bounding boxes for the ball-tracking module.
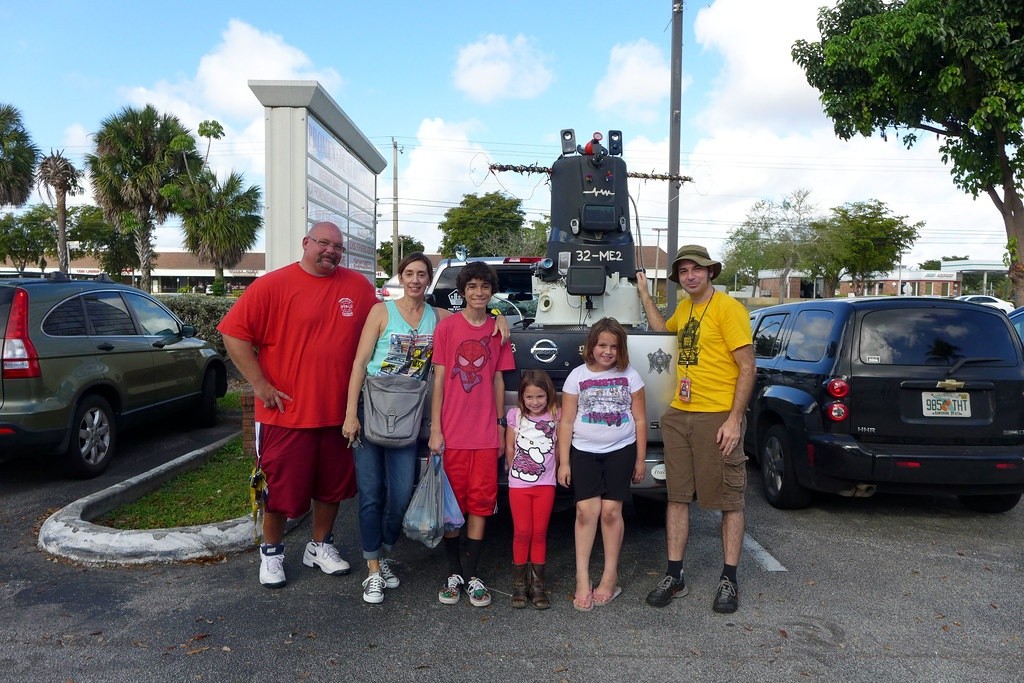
[608,130,622,155]
[560,129,576,154]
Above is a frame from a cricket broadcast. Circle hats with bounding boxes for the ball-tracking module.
[668,245,722,283]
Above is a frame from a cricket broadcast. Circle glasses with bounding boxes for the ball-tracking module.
[308,236,346,254]
[409,330,419,357]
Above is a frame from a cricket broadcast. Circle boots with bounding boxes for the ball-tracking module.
[511,564,528,609]
[529,563,550,610]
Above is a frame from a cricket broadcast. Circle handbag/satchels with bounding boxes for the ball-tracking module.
[361,376,430,446]
[402,451,465,549]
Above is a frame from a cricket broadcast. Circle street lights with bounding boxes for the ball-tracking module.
[652,227,668,296]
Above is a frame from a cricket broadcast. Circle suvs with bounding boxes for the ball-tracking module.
[0,271,230,480]
[746,296,1024,512]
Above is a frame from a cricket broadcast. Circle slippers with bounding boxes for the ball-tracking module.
[591,587,622,606]
[573,577,594,613]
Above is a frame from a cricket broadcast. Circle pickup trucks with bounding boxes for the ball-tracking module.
[426,255,680,500]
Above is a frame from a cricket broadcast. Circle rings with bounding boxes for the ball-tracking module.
[508,332,510,336]
[732,442,736,446]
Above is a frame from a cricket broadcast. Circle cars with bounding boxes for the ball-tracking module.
[954,294,1016,315]
[381,273,405,302]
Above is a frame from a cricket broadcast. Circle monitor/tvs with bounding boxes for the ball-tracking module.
[566,266,606,296]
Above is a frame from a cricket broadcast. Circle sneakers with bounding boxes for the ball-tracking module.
[260,543,288,589]
[367,559,400,589]
[646,569,688,608]
[713,576,738,613]
[465,577,492,607]
[438,574,464,605]
[362,574,386,604]
[302,534,351,576]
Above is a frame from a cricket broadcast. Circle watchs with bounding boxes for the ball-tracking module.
[497,417,508,428]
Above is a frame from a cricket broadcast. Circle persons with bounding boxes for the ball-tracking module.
[636,245,758,613]
[342,250,511,603]
[506,369,564,608]
[216,222,382,588]
[556,317,647,611]
[428,260,516,607]
